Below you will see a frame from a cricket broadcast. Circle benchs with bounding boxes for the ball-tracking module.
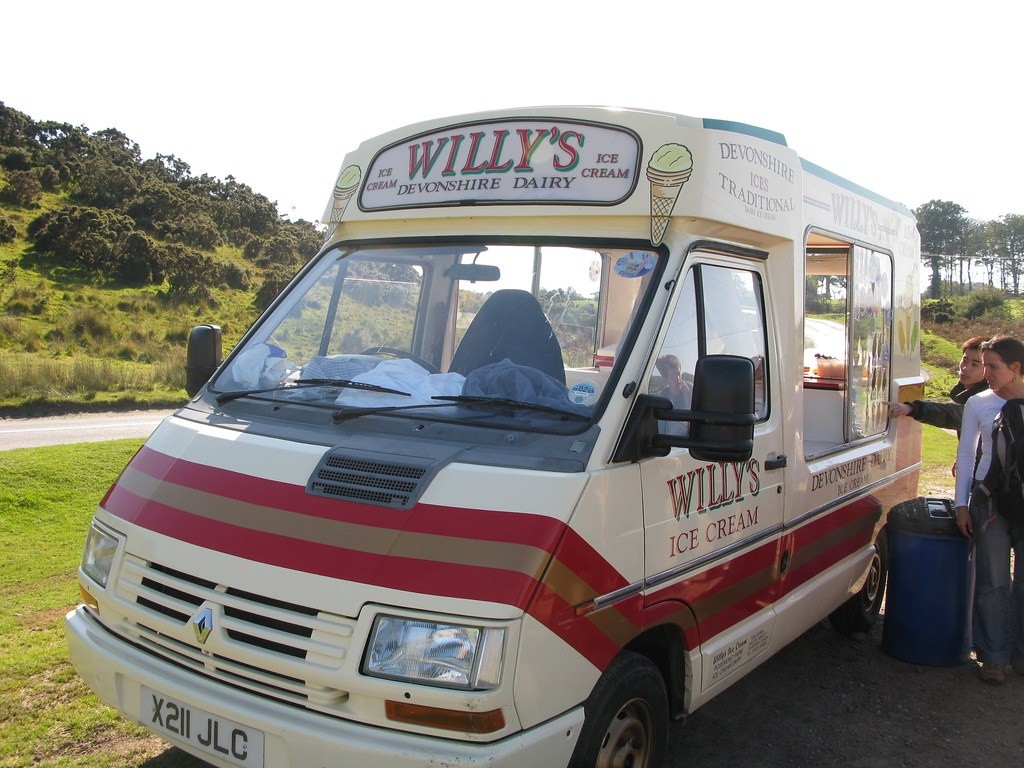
[798,377,848,442]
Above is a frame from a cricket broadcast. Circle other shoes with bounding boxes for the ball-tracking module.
[1011,661,1024,674]
[981,661,1006,685]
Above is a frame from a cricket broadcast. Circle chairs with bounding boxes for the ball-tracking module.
[447,289,567,387]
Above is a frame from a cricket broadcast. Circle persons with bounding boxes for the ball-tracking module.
[648,355,694,435]
[882,336,1024,684]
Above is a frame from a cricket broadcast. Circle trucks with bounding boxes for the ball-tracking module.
[64,107,924,768]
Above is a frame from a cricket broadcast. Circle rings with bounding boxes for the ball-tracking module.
[888,405,894,411]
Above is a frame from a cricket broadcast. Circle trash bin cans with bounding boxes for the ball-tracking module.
[880,498,976,666]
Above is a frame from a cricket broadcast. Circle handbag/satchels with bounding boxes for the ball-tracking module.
[262,356,298,388]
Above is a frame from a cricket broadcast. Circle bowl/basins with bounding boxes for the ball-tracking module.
[817,359,844,377]
